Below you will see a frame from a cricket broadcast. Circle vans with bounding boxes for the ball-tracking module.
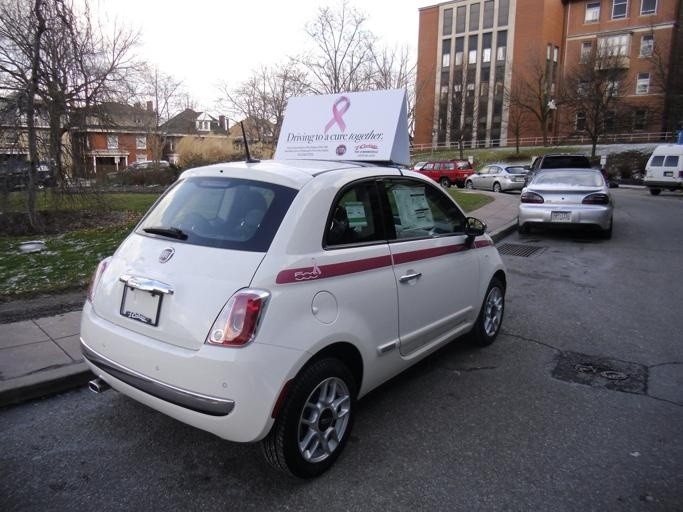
[0,159,54,191]
[129,159,170,170]
[642,141,682,195]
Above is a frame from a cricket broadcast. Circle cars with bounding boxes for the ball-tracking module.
[71,118,514,489]
[462,162,532,194]
[517,169,618,239]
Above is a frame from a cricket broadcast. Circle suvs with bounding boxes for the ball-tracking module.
[410,157,476,188]
[522,152,601,188]
